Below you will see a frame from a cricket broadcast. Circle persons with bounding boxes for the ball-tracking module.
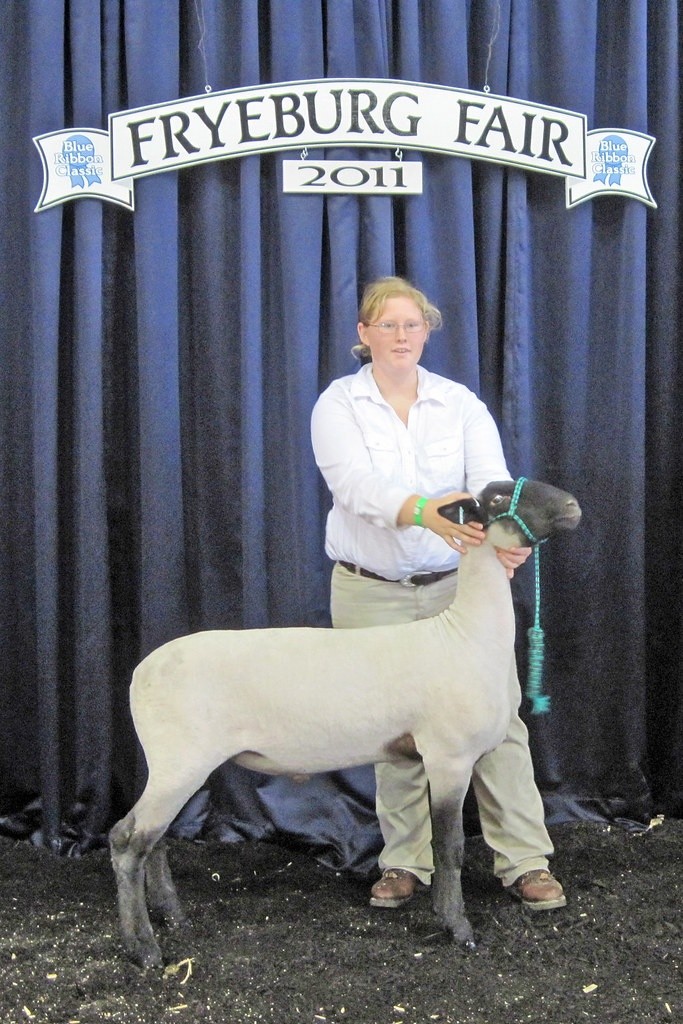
[311,278,567,910]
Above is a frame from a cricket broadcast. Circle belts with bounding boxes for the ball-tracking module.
[338,561,459,587]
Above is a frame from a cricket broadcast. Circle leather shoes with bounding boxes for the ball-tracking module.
[369,868,416,908]
[505,868,567,910]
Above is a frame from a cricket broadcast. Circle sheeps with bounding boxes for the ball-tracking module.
[108,480,581,967]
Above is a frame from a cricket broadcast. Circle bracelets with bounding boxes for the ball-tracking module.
[413,498,428,527]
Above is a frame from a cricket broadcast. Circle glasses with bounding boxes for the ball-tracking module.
[366,319,426,334]
